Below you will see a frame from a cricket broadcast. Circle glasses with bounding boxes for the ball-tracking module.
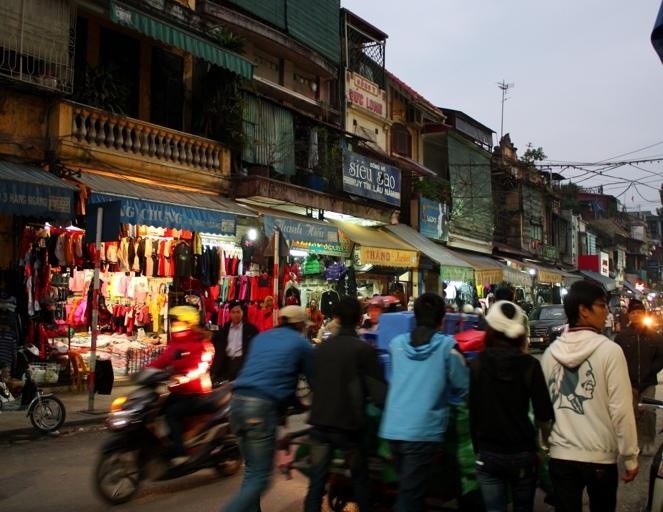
[594,302,608,309]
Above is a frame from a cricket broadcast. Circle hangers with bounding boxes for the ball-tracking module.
[65,218,84,231]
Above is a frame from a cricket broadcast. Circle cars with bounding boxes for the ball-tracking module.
[530,304,566,351]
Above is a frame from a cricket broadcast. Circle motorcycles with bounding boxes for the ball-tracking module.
[92,368,243,504]
[0,344,66,432]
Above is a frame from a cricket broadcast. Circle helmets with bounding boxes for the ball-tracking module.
[168,307,200,336]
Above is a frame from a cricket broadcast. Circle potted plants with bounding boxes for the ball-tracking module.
[207,83,294,177]
[213,28,250,54]
[294,104,343,193]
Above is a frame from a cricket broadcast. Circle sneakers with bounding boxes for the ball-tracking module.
[640,444,655,457]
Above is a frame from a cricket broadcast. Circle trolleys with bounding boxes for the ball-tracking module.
[277,350,539,511]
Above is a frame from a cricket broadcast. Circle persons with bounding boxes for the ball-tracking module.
[142,306,216,467]
[614,300,661,455]
[604,300,630,337]
[468,302,553,511]
[210,300,260,379]
[304,293,389,512]
[222,306,310,512]
[306,292,337,343]
[380,293,469,512]
[531,280,638,510]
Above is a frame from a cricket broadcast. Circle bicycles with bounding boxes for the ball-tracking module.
[638,396,662,512]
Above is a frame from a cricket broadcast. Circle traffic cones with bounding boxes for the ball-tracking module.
[88,208,103,411]
[273,231,280,328]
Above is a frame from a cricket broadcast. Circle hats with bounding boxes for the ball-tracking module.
[485,299,524,338]
[628,299,645,313]
[281,306,317,327]
